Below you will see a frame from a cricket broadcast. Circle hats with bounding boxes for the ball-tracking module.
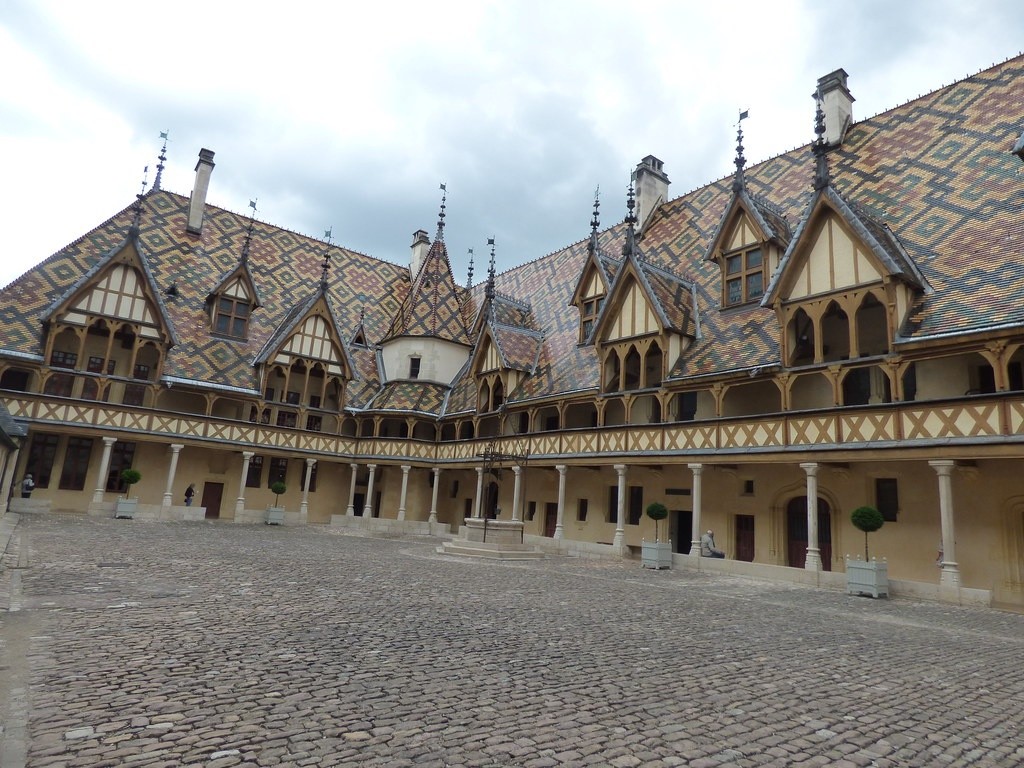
[26,474,32,479]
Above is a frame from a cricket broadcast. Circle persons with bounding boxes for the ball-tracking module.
[700,529,725,559]
[20,474,35,498]
[183,483,196,506]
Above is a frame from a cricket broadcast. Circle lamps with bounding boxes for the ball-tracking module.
[164,279,178,298]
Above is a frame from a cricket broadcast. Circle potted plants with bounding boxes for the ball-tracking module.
[114,469,141,519]
[641,503,672,570]
[845,505,890,599]
[264,481,287,525]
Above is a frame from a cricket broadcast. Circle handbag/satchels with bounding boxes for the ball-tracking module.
[26,482,33,490]
[184,499,187,502]
